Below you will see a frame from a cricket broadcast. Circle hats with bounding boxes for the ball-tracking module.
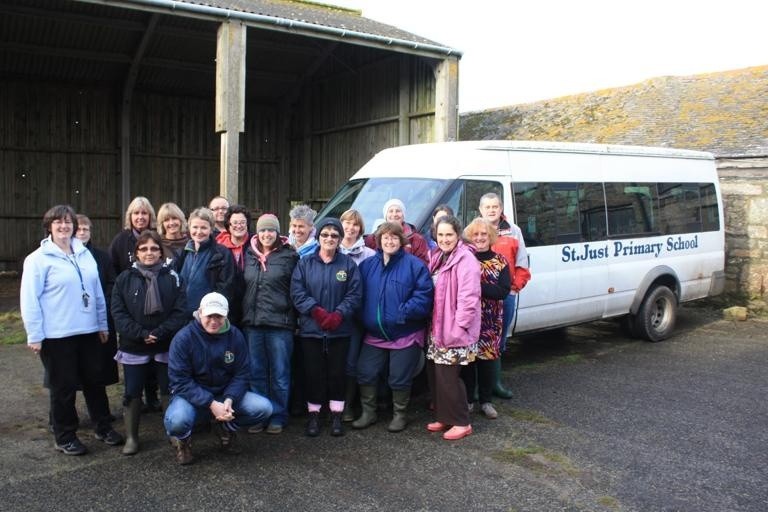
[254,212,283,237]
[199,289,231,319]
[313,215,346,244]
[381,197,408,222]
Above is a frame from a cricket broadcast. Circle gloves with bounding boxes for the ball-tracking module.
[310,306,346,332]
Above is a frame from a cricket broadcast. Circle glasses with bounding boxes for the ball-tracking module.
[139,245,160,254]
[211,205,230,214]
[53,216,74,228]
[381,234,401,244]
[471,231,490,239]
[227,220,250,228]
[75,227,92,235]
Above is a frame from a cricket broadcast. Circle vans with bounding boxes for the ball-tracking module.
[308,137,731,353]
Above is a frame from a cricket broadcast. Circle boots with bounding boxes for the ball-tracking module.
[118,399,147,458]
[351,384,383,430]
[490,354,513,400]
[340,379,356,423]
[386,386,414,433]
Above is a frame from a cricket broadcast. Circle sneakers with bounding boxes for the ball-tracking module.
[172,437,199,468]
[211,423,245,459]
[92,421,127,448]
[264,423,285,436]
[328,408,347,439]
[49,431,90,459]
[304,409,324,439]
[424,419,447,432]
[246,421,269,434]
[142,394,164,411]
[442,423,474,442]
[480,401,499,421]
[463,400,477,415]
